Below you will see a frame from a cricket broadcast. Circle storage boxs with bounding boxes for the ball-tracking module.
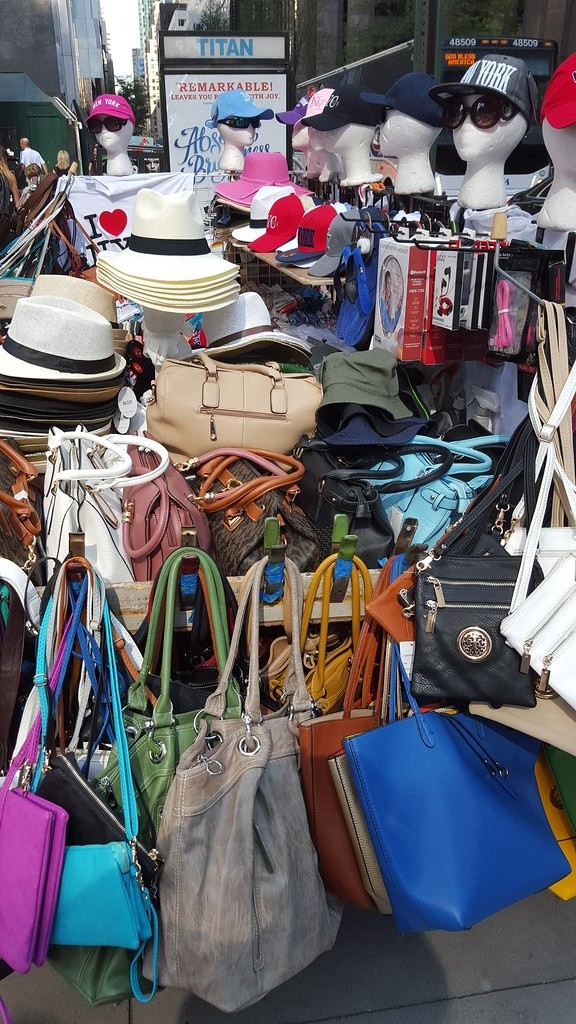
[374,231,566,366]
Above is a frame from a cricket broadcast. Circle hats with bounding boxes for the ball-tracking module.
[360,72,443,128]
[0,152,429,472]
[301,84,385,131]
[294,88,335,130]
[84,94,135,126]
[211,89,274,127]
[540,52,576,129]
[276,95,310,125]
[428,54,538,136]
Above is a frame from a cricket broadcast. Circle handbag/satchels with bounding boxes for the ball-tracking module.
[0,300,576,1024]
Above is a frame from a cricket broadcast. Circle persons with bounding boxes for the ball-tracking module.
[533,52,576,235]
[85,94,139,175]
[207,89,274,175]
[427,53,539,210]
[52,150,77,176]
[18,136,49,176]
[144,158,160,173]
[14,163,42,209]
[276,82,345,182]
[360,71,445,195]
[0,143,20,254]
[302,81,383,185]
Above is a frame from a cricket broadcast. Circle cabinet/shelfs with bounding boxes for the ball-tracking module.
[22,232,399,625]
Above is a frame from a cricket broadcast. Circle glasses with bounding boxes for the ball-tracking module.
[379,106,394,123]
[87,116,128,134]
[218,117,260,128]
[442,93,520,129]
